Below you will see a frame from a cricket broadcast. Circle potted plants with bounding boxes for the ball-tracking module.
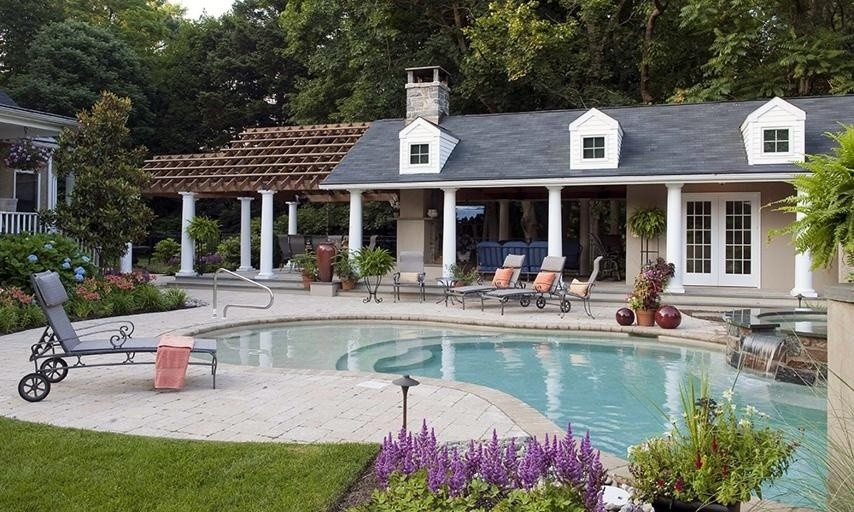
[289,248,321,292]
[447,262,484,288]
[329,246,367,291]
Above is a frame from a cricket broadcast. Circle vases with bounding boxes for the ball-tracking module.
[633,306,657,327]
[648,489,745,511]
[393,211,400,220]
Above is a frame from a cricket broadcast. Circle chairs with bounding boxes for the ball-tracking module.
[486,254,571,313]
[392,250,426,304]
[444,254,530,313]
[559,254,604,321]
[17,268,217,404]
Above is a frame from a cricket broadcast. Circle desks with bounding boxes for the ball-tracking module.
[435,276,462,308]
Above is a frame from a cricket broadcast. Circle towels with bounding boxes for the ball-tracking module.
[151,335,195,392]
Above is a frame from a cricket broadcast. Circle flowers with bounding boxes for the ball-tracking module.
[1,134,53,174]
[388,192,403,212]
[612,362,809,512]
[623,255,678,312]
